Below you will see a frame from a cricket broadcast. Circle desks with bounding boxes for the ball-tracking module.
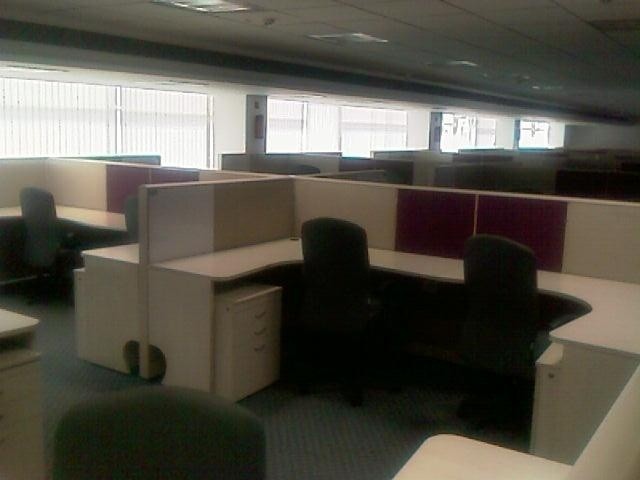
[1,309,49,353]
[1,202,139,376]
[153,235,639,463]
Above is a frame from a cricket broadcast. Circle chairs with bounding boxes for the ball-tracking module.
[297,218,391,407]
[454,234,537,423]
[52,383,268,479]
[19,186,88,306]
[123,189,160,243]
